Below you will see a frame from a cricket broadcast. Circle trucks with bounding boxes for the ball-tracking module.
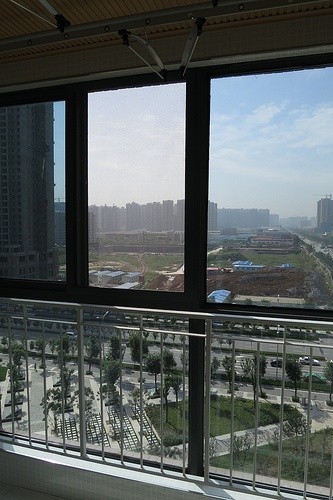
[207,267,231,275]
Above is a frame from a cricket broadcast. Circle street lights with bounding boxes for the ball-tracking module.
[276,325,279,380]
[96,311,109,360]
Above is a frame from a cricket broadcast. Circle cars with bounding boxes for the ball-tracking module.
[271,359,283,368]
[299,356,320,366]
[64,332,77,338]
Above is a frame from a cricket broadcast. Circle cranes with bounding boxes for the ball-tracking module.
[312,192,333,198]
[55,197,65,202]
